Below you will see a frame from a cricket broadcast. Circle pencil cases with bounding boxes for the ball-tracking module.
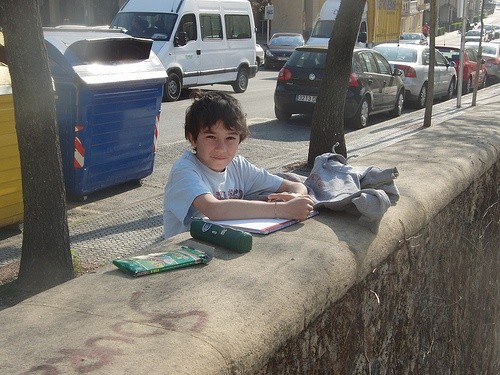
[190,221,252,253]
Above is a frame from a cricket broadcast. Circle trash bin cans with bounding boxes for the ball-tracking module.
[43,25,169,201]
[0,31,57,230]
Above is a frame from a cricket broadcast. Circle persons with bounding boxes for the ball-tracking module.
[162,90,317,240]
[125,17,150,39]
[422,22,430,38]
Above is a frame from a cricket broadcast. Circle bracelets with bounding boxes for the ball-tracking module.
[275,201,278,218]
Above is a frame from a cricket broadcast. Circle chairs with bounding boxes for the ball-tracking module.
[408,35,411,39]
[386,49,399,60]
[416,35,420,39]
[313,54,327,68]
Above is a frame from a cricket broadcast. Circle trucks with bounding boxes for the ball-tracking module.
[305,0,425,50]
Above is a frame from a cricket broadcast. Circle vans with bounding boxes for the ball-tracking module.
[107,0,259,103]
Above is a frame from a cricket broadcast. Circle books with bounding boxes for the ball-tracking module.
[202,210,319,235]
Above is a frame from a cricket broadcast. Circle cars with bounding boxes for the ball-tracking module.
[367,42,459,107]
[399,32,430,46]
[426,24,500,97]
[272,44,406,130]
[262,33,305,69]
[256,41,267,74]
